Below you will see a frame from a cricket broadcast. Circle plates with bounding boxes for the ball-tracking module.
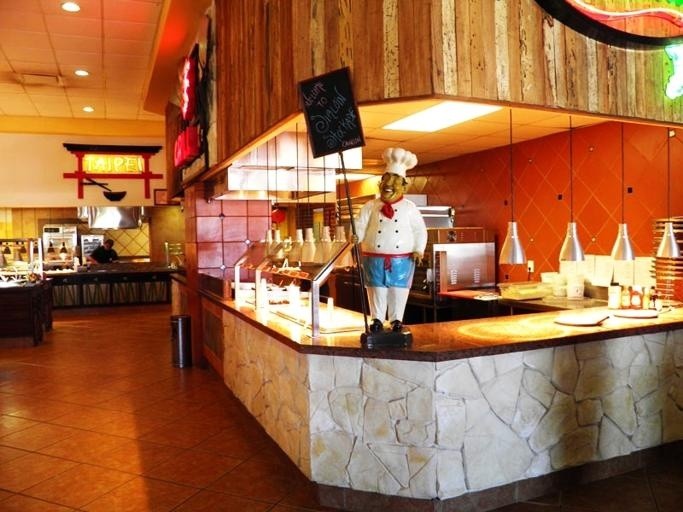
[649,215,683,307]
[614,310,658,318]
[555,317,609,326]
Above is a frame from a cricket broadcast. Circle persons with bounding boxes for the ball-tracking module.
[347,147,428,332]
[90,238,118,264]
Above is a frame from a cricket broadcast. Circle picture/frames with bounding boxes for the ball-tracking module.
[154,188,180,205]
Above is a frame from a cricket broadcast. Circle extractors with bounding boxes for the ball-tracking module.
[87,205,138,230]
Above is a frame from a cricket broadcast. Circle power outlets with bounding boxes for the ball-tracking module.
[526,260,534,272]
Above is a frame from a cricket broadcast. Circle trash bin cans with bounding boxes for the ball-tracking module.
[169,315,192,368]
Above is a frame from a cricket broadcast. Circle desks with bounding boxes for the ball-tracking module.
[0,275,56,347]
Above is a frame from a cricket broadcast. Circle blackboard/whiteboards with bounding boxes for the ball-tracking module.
[298,66,366,159]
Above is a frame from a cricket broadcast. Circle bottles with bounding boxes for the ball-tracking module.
[607,282,664,311]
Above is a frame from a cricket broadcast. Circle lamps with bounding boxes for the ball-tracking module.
[260,120,352,268]
[557,111,585,262]
[654,126,680,259]
[495,106,527,264]
[609,121,634,261]
